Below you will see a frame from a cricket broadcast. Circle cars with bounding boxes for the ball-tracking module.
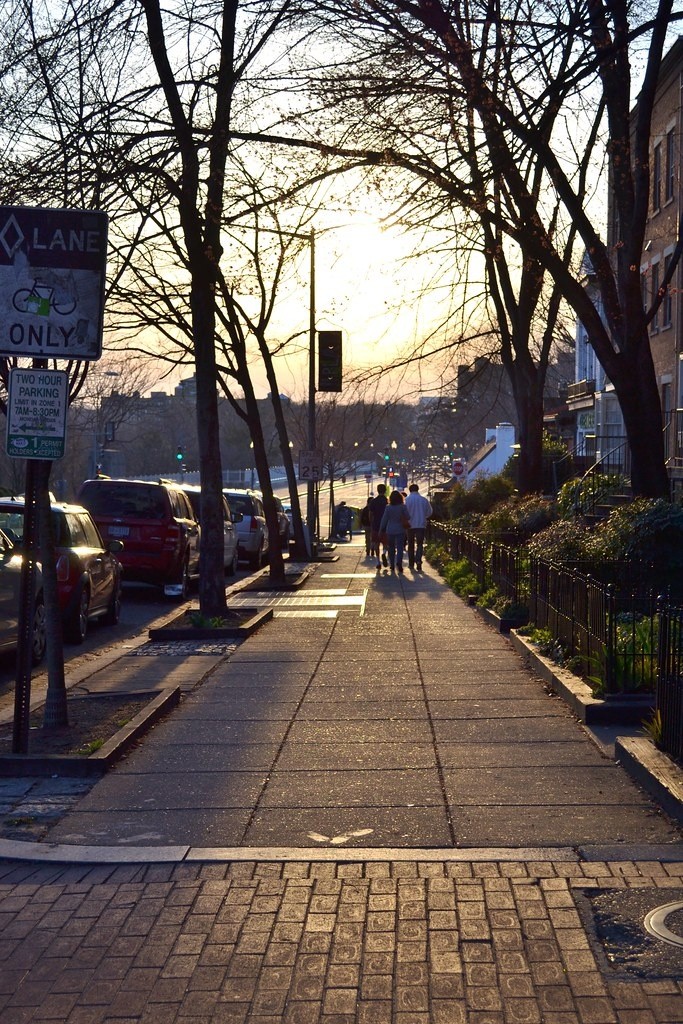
[260,493,292,550]
[0,528,47,667]
[280,501,296,541]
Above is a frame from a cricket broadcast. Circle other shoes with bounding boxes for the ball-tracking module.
[396,563,403,572]
[408,565,413,568]
[417,564,421,570]
[390,566,394,569]
[376,564,381,570]
[382,554,388,567]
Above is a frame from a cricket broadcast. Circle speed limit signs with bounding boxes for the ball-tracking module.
[298,450,323,481]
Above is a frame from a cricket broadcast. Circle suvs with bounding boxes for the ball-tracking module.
[0,495,124,645]
[75,475,200,605]
[178,484,244,577]
[222,488,269,571]
[17,491,57,505]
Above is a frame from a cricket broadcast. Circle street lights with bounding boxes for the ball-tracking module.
[390,440,397,491]
[427,442,432,496]
[354,442,359,480]
[410,442,416,484]
[370,443,374,471]
[248,441,254,489]
[444,443,448,481]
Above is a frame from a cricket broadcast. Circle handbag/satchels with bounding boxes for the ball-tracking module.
[400,505,411,530]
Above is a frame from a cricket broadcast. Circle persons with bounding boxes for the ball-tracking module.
[359,483,433,574]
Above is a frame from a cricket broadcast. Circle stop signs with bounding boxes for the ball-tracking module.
[452,461,465,478]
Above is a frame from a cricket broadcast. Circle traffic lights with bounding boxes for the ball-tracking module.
[177,446,183,460]
[382,448,390,461]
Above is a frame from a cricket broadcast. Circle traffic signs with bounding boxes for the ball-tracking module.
[4,367,69,460]
